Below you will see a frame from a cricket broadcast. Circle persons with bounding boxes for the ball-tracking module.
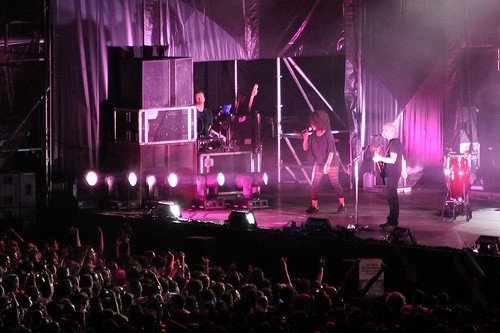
[194,90,215,135]
[302,108,346,215]
[0,224,500,333]
[373,123,403,226]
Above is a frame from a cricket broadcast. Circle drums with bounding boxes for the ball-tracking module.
[443,151,470,201]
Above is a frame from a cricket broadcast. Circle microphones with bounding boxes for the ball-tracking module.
[301,127,312,136]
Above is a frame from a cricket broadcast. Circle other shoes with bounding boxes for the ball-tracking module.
[379,222,397,228]
[306,206,320,215]
[337,204,346,214]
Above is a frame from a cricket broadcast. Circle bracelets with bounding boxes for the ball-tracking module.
[324,162,329,166]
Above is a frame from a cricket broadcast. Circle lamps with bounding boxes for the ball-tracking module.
[248,172,269,186]
[159,174,178,187]
[228,210,257,227]
[304,217,332,232]
[120,172,138,186]
[388,227,417,245]
[142,175,157,187]
[475,235,500,253]
[159,201,182,219]
[84,171,114,187]
[202,173,225,188]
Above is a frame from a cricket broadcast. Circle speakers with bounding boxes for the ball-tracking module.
[0,174,19,209]
[136,58,171,110]
[20,172,37,208]
[73,194,96,210]
[303,217,332,233]
[126,143,167,208]
[474,236,500,255]
[167,143,195,206]
[370,134,381,139]
[390,226,417,246]
[157,201,181,219]
[171,57,194,107]
[229,210,257,228]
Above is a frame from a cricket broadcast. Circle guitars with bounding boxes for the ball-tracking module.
[374,150,383,180]
